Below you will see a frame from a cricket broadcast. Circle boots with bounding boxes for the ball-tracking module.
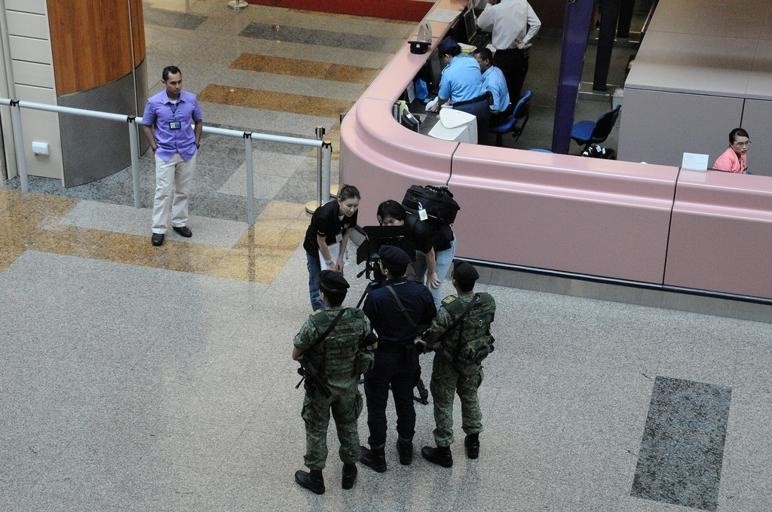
[360,444,389,474]
[421,444,455,469]
[465,432,482,460]
[342,462,359,490]
[295,469,327,495]
[396,438,414,467]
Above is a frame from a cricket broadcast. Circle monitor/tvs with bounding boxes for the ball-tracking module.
[428,107,478,144]
[401,108,419,133]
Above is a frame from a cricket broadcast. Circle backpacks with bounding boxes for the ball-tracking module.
[403,183,461,225]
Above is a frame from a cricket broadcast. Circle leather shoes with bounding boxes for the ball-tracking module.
[173,226,192,237]
[151,232,164,246]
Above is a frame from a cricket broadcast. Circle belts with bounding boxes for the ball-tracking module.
[453,94,486,107]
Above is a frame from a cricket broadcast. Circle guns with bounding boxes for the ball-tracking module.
[295,352,331,401]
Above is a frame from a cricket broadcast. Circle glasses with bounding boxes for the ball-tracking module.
[736,141,752,147]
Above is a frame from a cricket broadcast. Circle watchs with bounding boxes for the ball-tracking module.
[150,145,160,152]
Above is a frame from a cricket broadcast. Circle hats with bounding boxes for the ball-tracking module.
[378,244,410,270]
[316,270,351,294]
[452,260,480,282]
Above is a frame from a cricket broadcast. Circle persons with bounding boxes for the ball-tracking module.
[302,185,361,313]
[141,66,202,245]
[291,270,376,495]
[474,49,512,126]
[711,128,750,176]
[414,261,497,467]
[359,245,440,473]
[429,37,490,137]
[476,0,541,110]
[376,200,455,314]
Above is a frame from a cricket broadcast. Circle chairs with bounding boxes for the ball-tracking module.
[485,90,534,145]
[571,105,623,155]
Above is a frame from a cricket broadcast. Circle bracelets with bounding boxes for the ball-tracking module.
[195,141,201,150]
[324,259,334,266]
[436,101,441,108]
